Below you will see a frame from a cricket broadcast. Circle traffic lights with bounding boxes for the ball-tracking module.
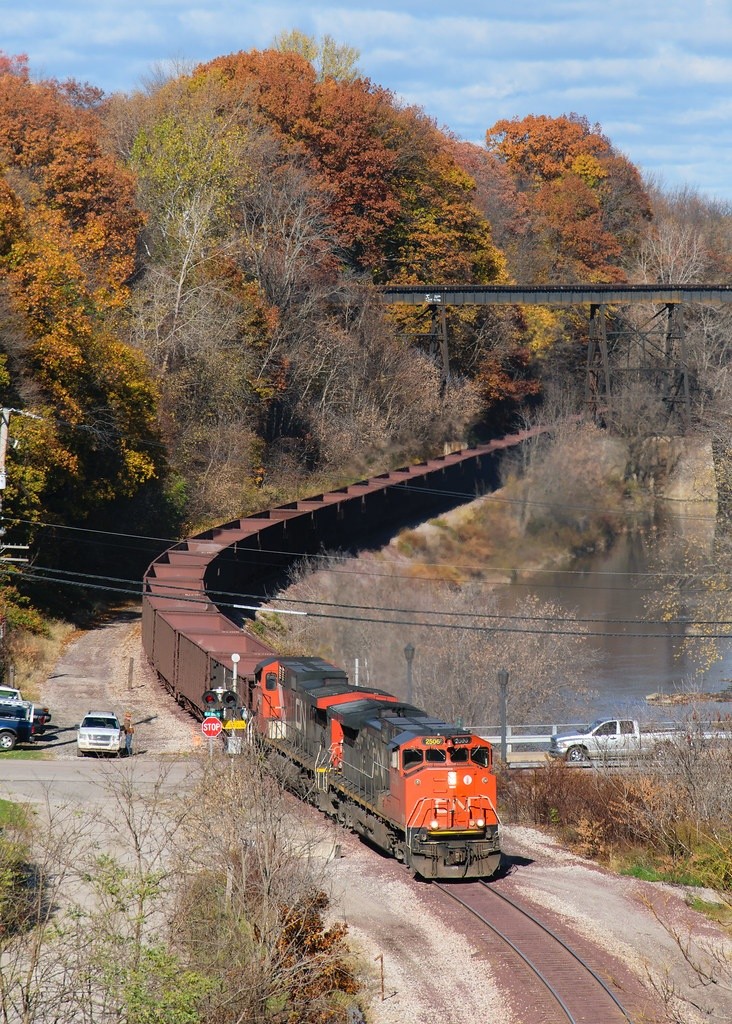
[222,690,240,708]
[201,692,218,706]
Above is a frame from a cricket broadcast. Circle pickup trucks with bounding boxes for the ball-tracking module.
[550,717,690,768]
[0,686,52,750]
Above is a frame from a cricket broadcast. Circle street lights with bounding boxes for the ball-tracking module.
[496,667,512,792]
[403,640,417,705]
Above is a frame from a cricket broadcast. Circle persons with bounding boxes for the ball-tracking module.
[124,711,135,755]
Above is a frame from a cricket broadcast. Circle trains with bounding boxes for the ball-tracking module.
[142,408,614,881]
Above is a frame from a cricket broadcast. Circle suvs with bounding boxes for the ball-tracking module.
[77,709,122,757]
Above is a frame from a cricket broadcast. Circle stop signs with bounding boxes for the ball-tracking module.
[202,717,223,738]
